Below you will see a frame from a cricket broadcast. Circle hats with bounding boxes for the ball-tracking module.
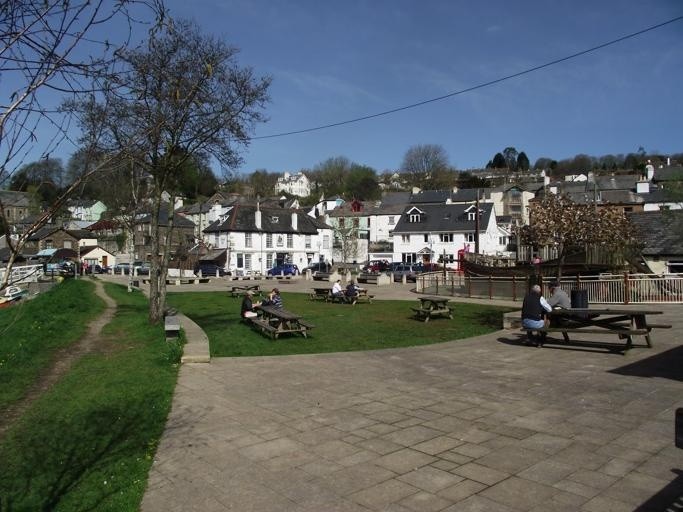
[247,290,256,295]
[547,281,561,288]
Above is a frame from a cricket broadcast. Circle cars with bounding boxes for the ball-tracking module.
[102,261,152,275]
[268,264,300,279]
[86,265,103,274]
[194,262,232,278]
[302,262,331,275]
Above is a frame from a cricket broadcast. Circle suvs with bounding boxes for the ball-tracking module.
[386,262,423,283]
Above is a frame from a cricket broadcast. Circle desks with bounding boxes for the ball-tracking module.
[415,296,454,323]
[227,284,374,339]
[537,308,663,356]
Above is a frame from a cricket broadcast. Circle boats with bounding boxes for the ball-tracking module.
[0,286,32,304]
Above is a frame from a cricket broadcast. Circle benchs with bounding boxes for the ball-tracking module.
[409,306,433,313]
[560,321,672,328]
[438,304,457,310]
[164,316,180,344]
[520,326,649,335]
[143,275,293,286]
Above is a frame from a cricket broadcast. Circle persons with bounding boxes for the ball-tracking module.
[520,285,553,347]
[535,281,572,340]
[81,260,88,275]
[264,287,282,322]
[344,280,363,305]
[331,280,343,304]
[240,290,262,324]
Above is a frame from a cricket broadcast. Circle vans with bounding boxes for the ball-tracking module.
[363,259,389,273]
